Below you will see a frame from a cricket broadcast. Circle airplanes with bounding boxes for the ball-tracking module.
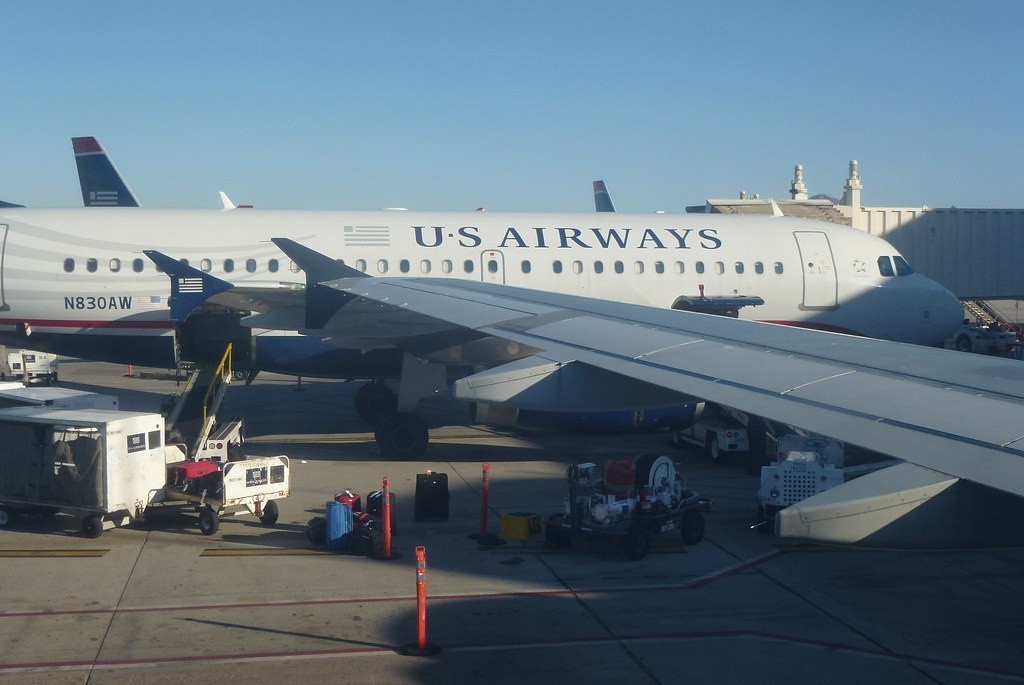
[0,135,1024,551]
